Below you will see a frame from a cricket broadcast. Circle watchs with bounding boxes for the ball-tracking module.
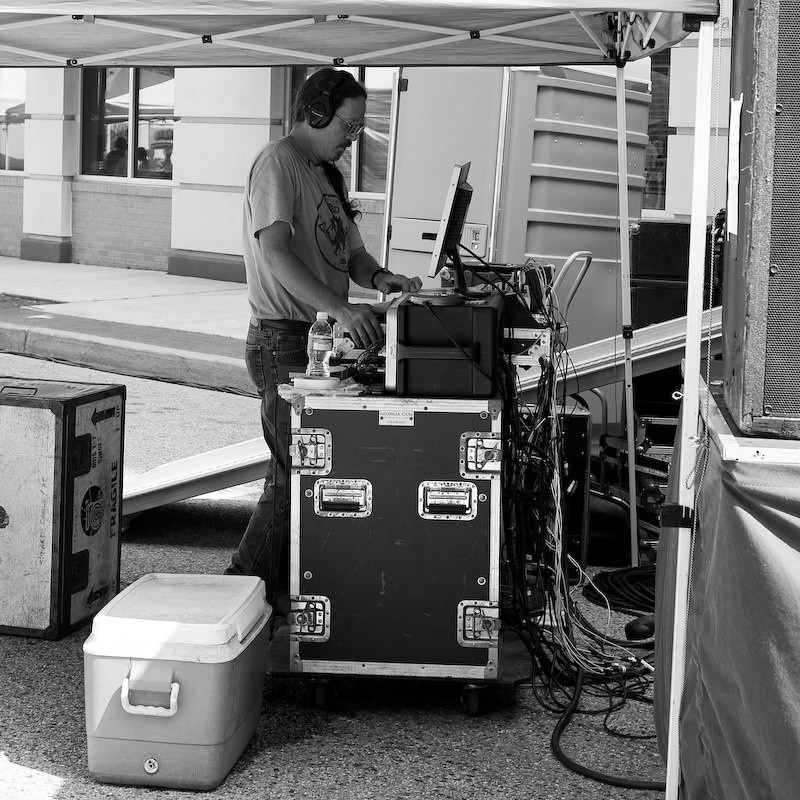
[370,267,394,289]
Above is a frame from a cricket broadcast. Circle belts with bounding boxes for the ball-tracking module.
[261,318,334,333]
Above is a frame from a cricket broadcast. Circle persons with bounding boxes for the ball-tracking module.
[223,67,424,610]
[104,136,151,173]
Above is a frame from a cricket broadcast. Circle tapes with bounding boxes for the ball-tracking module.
[294,376,340,389]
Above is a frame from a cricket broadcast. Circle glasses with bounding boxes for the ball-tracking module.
[334,113,364,135]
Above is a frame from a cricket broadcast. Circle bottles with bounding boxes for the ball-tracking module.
[305,311,333,379]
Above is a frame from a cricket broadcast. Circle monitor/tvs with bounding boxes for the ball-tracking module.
[427,159,492,300]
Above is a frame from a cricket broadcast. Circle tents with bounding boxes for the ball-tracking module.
[0,0,722,800]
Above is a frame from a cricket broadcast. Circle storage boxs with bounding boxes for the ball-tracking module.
[84,574,273,792]
[0,376,127,642]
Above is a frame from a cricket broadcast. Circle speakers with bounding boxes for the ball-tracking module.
[520,398,593,586]
[625,219,712,419]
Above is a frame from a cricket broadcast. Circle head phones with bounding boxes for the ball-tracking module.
[306,71,350,129]
